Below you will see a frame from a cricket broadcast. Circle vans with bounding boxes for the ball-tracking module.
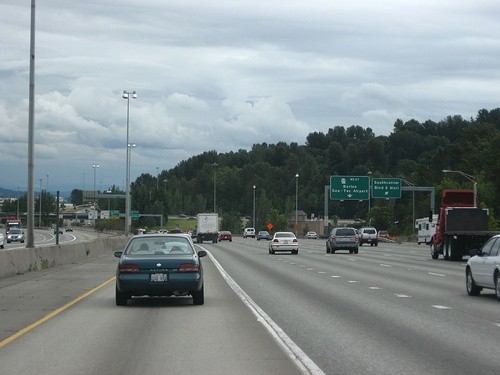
[243,228,256,238]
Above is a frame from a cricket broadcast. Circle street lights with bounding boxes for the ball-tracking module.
[252,185,256,230]
[294,173,299,225]
[441,169,478,186]
[93,163,100,227]
[123,90,137,236]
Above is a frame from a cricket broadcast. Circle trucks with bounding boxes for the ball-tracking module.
[5,219,22,232]
[194,213,218,244]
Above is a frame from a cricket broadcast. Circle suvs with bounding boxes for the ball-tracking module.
[358,228,379,247]
[464,234,500,299]
[325,227,359,255]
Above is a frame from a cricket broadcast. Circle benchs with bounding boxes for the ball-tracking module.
[135,250,186,254]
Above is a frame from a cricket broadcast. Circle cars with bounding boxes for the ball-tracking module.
[6,229,25,243]
[308,232,317,239]
[268,231,299,255]
[218,230,233,242]
[256,230,269,241]
[113,233,207,306]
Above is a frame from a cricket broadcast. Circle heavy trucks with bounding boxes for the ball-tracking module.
[426,182,500,261]
